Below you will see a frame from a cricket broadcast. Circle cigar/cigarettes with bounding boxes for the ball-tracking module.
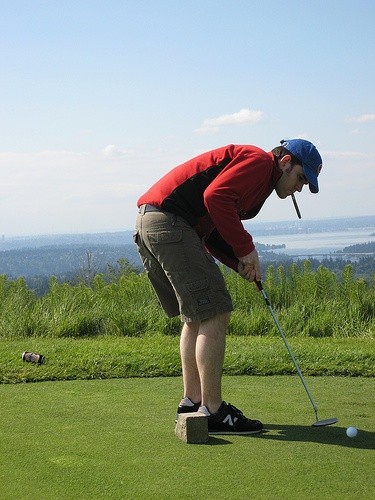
[291,193,301,219]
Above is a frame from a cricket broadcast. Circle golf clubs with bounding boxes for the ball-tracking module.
[253,273,338,426]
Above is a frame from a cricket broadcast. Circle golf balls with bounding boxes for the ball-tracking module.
[346,427,359,438]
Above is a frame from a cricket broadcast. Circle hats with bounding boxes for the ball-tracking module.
[280,139,322,193]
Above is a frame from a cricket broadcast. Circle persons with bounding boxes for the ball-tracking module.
[132,139,322,435]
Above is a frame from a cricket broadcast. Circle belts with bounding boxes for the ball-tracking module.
[139,204,162,213]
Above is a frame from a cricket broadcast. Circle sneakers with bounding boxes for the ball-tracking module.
[175,396,202,422]
[197,401,263,434]
